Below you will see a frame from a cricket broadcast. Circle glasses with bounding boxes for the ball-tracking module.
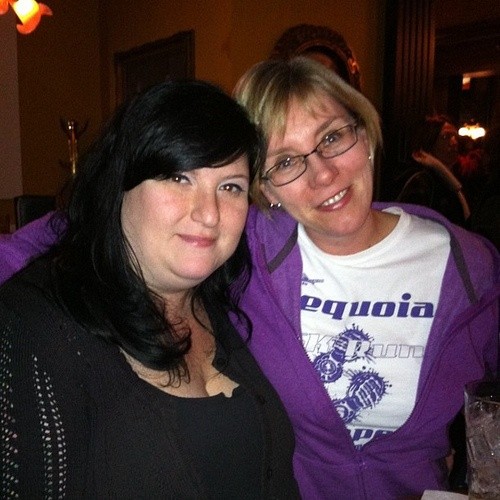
[261,122,361,187]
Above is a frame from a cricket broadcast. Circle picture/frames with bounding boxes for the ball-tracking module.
[115,26,196,124]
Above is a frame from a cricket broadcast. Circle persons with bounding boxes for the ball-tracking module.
[400,118,500,250]
[0,79,302,500]
[0,56,500,500]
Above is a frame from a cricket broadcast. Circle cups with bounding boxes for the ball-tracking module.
[464,379,500,500]
